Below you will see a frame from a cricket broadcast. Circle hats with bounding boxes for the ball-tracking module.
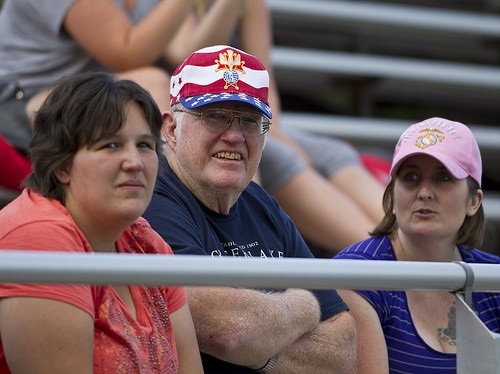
[166,44,274,120]
[390,117,482,189]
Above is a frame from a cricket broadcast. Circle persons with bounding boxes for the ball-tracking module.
[0,0,261,189]
[331,116,500,374]
[116,0,391,255]
[0,73,206,374]
[139,44,357,374]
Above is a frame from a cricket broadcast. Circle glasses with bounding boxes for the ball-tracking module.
[174,107,273,138]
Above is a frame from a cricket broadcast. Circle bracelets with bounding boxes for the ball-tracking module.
[260,353,278,373]
